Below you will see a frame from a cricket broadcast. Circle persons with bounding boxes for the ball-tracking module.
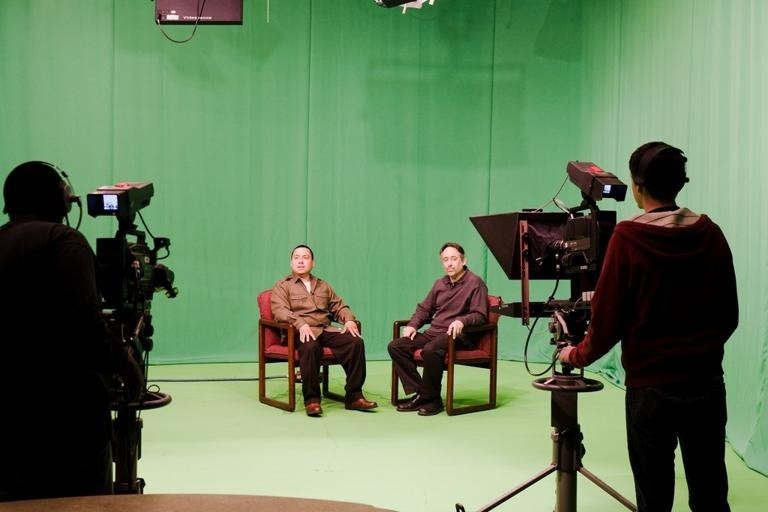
[387,241,490,416]
[558,140,740,512]
[0,160,147,503]
[270,244,379,415]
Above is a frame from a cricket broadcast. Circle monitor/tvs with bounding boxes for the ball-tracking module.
[154,0,244,25]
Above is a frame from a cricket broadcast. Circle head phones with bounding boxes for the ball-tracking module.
[4,161,78,215]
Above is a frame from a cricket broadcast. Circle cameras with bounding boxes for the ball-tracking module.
[567,160,628,202]
[87,180,154,218]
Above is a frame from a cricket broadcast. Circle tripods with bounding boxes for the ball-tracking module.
[455,345,639,512]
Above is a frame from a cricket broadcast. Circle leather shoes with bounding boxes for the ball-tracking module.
[417,401,444,417]
[397,393,422,411]
[304,399,323,418]
[345,398,377,410]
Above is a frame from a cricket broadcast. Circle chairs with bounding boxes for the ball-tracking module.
[257,289,362,412]
[390,295,502,415]
